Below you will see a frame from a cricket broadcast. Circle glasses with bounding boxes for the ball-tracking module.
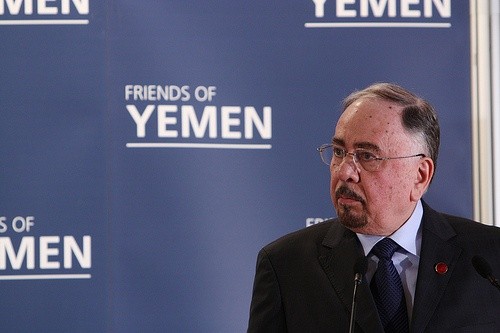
[316,143,426,176]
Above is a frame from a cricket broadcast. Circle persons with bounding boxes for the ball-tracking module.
[246,82,500,333]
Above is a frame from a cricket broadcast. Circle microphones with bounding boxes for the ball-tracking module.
[349,255,368,333]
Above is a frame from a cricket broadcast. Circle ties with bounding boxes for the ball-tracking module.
[371,237,410,333]
